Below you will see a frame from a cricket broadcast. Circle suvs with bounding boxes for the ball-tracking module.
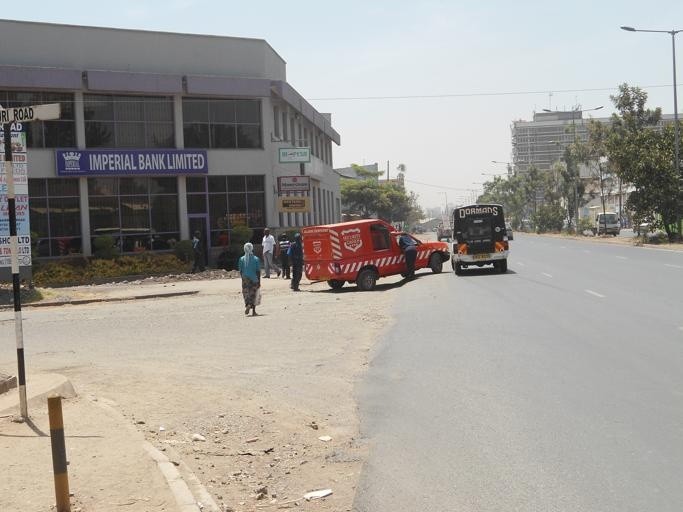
[436,220,453,242]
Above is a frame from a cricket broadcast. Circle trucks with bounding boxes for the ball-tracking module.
[300,218,451,291]
[588,204,620,237]
[449,202,510,276]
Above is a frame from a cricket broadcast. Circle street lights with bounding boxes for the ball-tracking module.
[473,160,528,189]
[618,25,683,192]
[542,106,606,233]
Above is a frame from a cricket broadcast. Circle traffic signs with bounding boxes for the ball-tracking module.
[0,103,60,124]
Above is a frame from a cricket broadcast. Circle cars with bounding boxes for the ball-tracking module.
[504,222,513,240]
[633,217,656,233]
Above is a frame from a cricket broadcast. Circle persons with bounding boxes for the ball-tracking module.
[279,233,292,279]
[288,233,303,291]
[399,232,417,280]
[261,227,281,278]
[191,232,205,274]
[219,232,227,240]
[620,218,626,228]
[238,242,261,316]
